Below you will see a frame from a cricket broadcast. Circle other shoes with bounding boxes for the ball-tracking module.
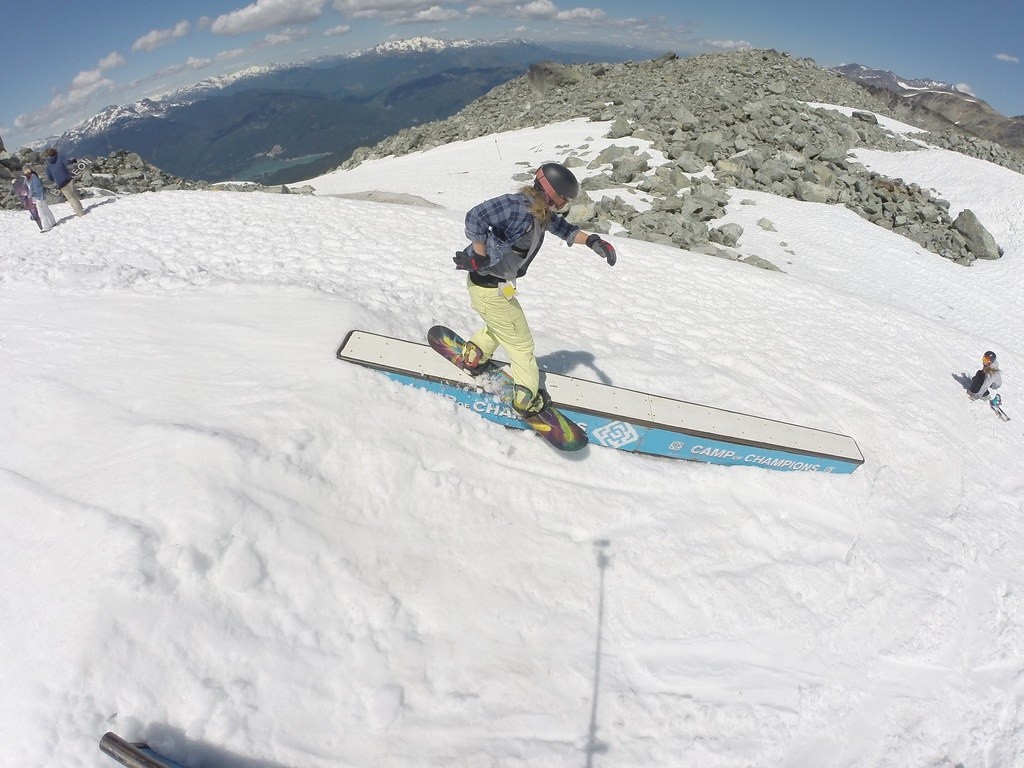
[512,389,550,417]
[40,230,47,233]
[51,224,56,226]
[462,341,490,376]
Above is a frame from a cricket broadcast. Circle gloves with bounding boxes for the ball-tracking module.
[453,250,490,273]
[586,234,616,267]
[72,159,77,163]
[12,179,17,184]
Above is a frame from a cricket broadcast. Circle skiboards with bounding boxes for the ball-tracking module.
[967,377,1011,421]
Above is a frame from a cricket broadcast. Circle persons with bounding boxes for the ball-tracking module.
[966,351,1002,402]
[11,166,57,232]
[453,162,617,418]
[44,149,84,216]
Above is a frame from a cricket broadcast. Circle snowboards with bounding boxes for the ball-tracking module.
[427,324,590,454]
[11,178,43,230]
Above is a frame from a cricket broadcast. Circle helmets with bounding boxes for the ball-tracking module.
[534,163,578,198]
[983,351,996,363]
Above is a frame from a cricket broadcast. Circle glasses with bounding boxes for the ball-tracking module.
[554,200,570,213]
[24,168,31,175]
[982,357,991,364]
